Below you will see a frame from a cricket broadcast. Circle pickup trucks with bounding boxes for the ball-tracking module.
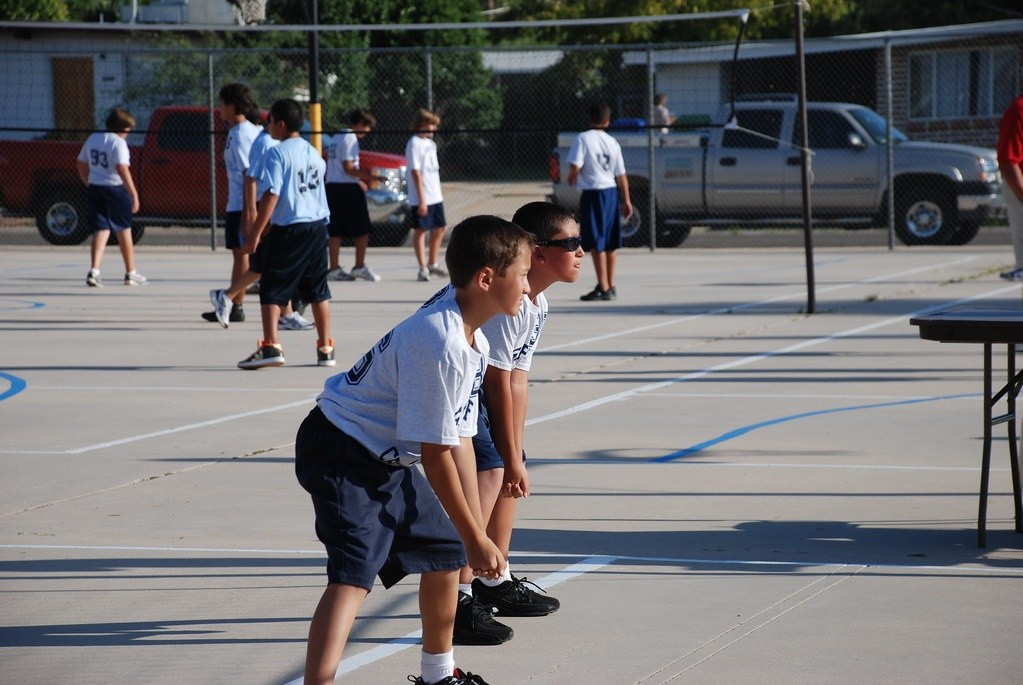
[548,99,1009,245]
[2,102,411,247]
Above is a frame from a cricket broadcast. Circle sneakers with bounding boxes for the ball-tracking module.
[453,598,514,646]
[414,668,490,685]
[278,310,316,331]
[317,338,337,367]
[238,339,286,370]
[210,289,233,329]
[471,573,560,616]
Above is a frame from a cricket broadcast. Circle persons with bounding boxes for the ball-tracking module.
[327,106,389,283]
[294,214,533,685]
[654,91,675,126]
[416,201,584,646]
[998,94,1023,282]
[566,101,633,302]
[405,108,451,281]
[201,83,337,370]
[77,106,148,289]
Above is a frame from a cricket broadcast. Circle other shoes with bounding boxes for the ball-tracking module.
[87,271,104,287]
[201,304,246,322]
[125,272,148,286]
[418,266,450,281]
[327,267,356,281]
[580,285,618,301]
[351,266,381,282]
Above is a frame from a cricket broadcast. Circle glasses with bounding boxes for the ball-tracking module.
[536,236,583,253]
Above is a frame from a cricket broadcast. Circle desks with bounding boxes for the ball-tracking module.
[909,305,1023,549]
